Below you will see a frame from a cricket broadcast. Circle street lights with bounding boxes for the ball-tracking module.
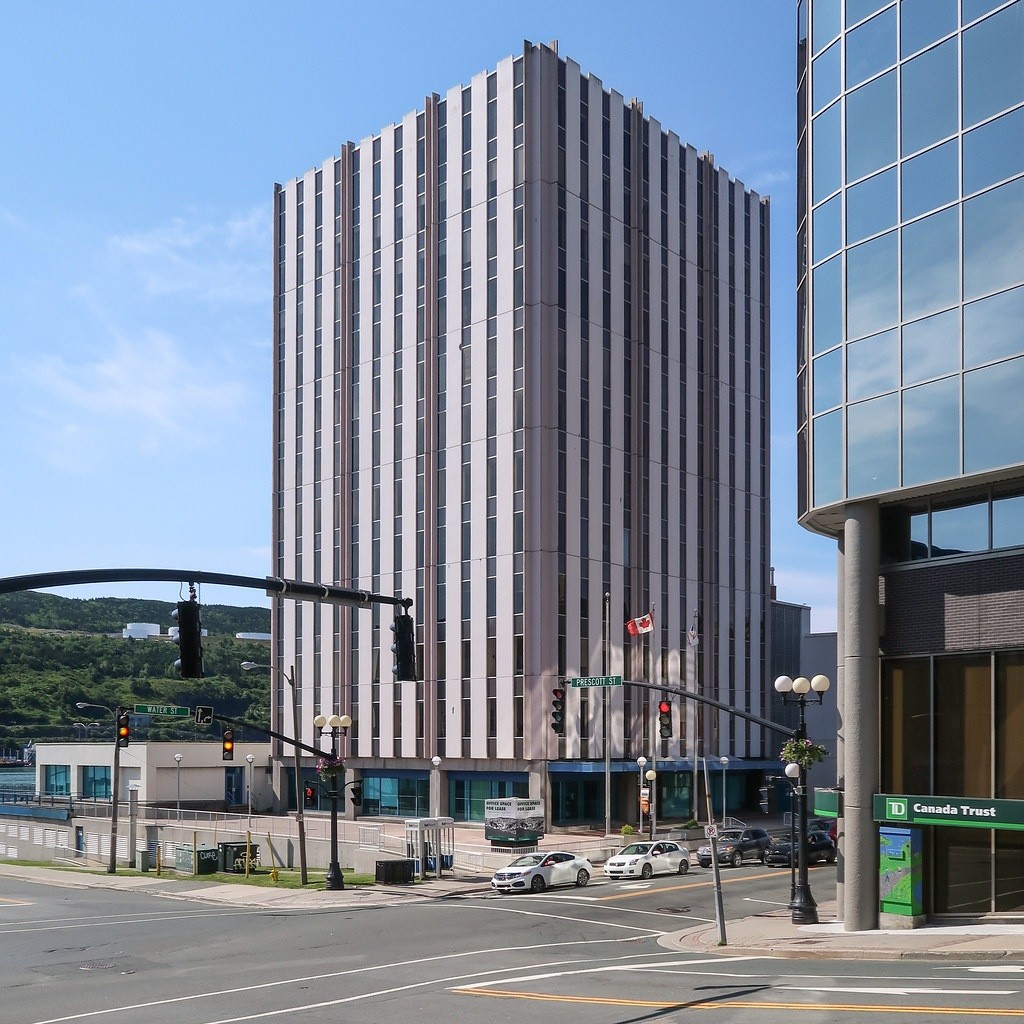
[636,756,647,832]
[245,753,255,827]
[174,753,183,821]
[313,715,353,890]
[430,756,443,817]
[784,763,800,910]
[72,702,121,872]
[720,756,729,829]
[774,674,830,925]
[240,661,309,886]
[645,770,657,840]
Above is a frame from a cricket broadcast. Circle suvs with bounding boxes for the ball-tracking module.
[695,825,774,867]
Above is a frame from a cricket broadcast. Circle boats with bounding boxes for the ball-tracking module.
[0,747,24,767]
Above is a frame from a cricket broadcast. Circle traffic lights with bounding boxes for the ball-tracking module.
[222,730,233,761]
[117,715,129,747]
[306,787,315,806]
[390,615,417,682]
[350,788,361,806]
[171,601,205,679]
[758,788,768,814]
[551,688,564,733]
[659,701,672,738]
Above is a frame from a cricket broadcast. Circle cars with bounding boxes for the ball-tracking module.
[764,817,837,869]
[603,840,691,881]
[490,850,594,896]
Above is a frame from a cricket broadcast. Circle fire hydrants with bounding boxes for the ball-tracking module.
[269,869,281,881]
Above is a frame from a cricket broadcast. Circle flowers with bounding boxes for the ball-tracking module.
[780,737,830,769]
[315,757,345,782]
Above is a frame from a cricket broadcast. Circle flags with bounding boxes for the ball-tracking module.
[625,609,654,637]
[688,624,700,648]
